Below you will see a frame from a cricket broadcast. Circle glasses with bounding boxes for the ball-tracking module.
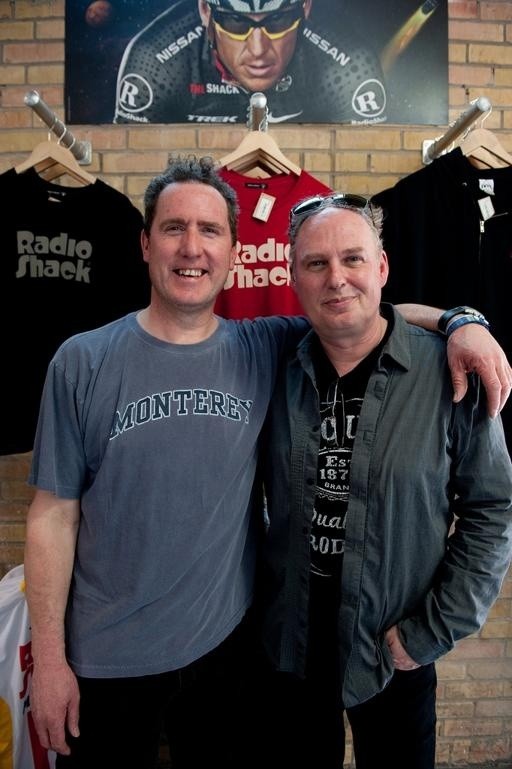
[207,4,305,40]
[289,193,375,226]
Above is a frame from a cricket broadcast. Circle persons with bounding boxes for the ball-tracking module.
[24,165,512,769]
[112,0,385,124]
[250,194,512,769]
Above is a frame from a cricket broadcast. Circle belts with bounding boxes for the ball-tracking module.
[299,566,333,595]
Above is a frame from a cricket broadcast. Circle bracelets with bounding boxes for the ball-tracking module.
[446,316,490,335]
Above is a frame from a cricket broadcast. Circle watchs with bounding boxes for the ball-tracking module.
[438,306,486,335]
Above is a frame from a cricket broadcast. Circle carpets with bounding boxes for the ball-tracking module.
[213,106,304,181]
[442,95,511,167]
[10,116,99,188]
